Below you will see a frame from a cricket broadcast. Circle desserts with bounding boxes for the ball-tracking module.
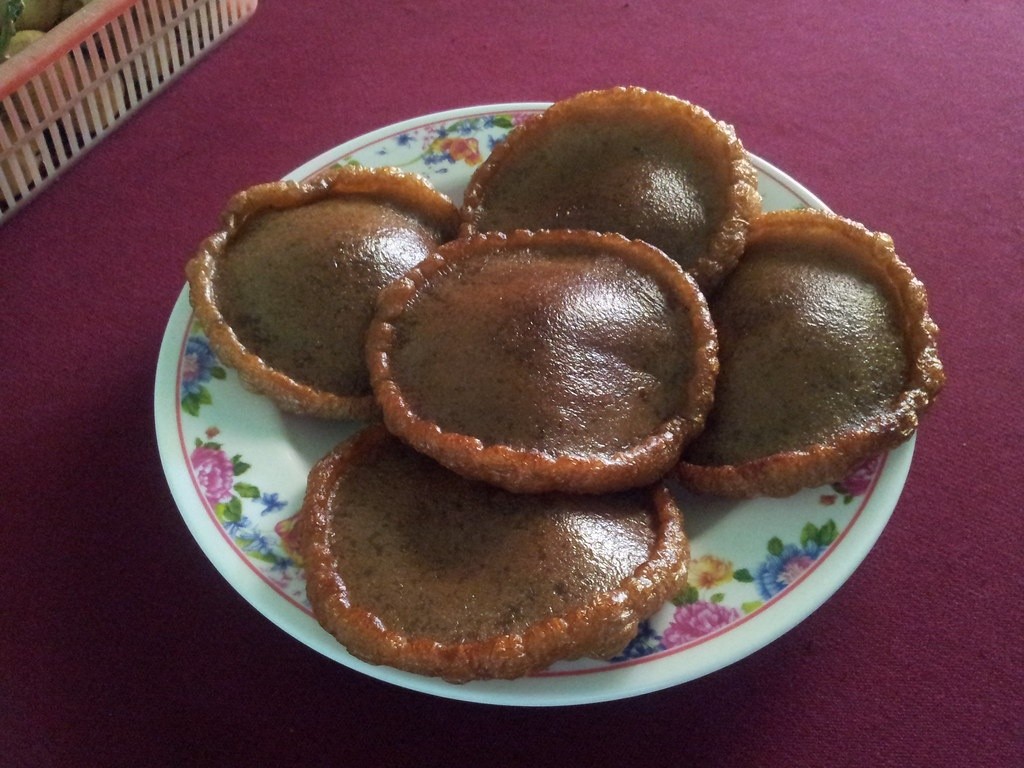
[187,83,947,681]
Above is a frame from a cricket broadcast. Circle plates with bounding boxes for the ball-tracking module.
[155,102,917,707]
